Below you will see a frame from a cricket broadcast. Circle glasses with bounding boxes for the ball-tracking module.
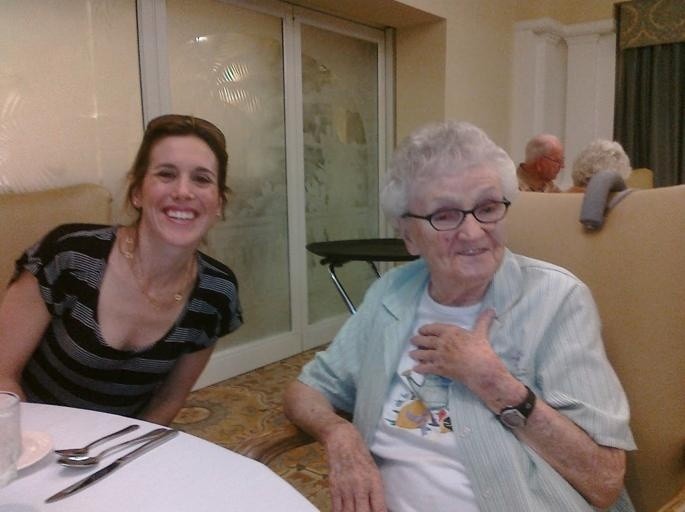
[402,195,512,233]
[540,153,567,167]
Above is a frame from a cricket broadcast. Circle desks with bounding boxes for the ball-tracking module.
[304,237,421,318]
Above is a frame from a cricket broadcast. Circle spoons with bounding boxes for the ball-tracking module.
[56,429,168,469]
[51,425,138,459]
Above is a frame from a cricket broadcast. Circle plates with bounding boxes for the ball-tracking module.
[8,428,54,474]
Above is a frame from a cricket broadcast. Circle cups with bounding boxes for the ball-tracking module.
[0,390,24,465]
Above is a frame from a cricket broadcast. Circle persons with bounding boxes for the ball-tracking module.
[0,113,245,425]
[515,133,564,191]
[569,142,630,192]
[283,119,633,512]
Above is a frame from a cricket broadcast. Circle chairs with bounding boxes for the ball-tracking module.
[0,181,114,308]
[230,176,685,510]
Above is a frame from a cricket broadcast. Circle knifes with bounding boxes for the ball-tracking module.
[44,429,178,505]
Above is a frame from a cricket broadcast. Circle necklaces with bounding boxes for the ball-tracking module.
[125,234,195,312]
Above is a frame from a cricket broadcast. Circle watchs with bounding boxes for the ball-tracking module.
[499,385,534,430]
